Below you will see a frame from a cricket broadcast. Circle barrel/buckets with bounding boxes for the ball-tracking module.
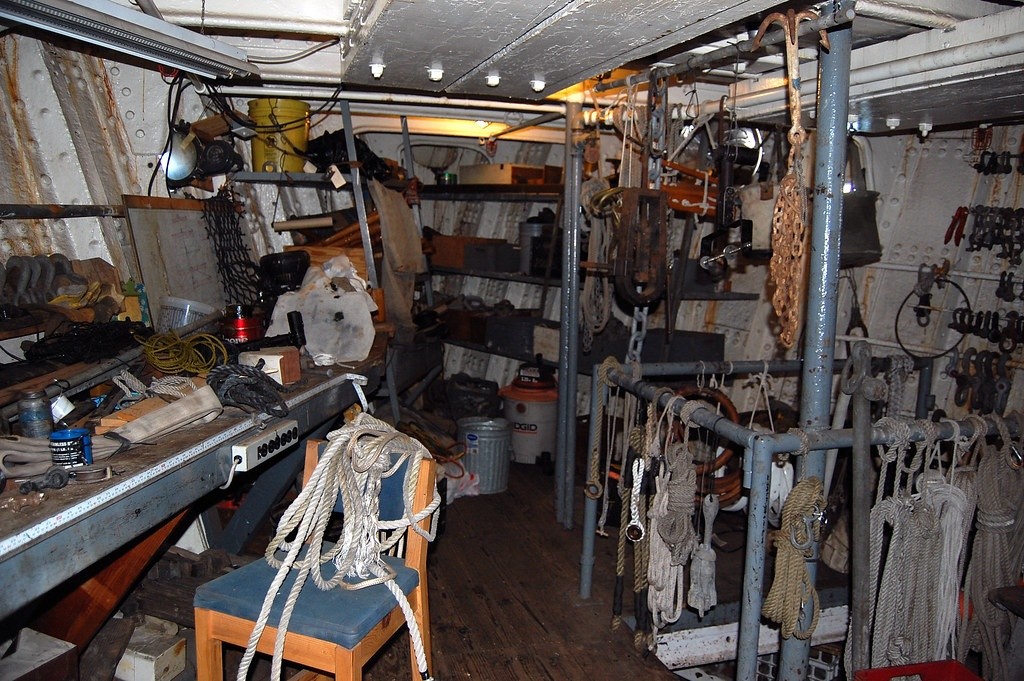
[500,386,559,464]
[457,417,513,494]
[247,98,310,172]
[157,295,215,340]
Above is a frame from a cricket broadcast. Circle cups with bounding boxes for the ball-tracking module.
[49,429,92,469]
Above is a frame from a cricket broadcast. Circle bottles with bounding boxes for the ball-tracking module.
[17,388,53,438]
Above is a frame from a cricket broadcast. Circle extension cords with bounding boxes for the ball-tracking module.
[232,420,299,472]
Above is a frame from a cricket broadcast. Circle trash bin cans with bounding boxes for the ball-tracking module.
[448,372,500,423]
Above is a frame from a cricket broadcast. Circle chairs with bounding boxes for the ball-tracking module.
[194,437,437,680]
